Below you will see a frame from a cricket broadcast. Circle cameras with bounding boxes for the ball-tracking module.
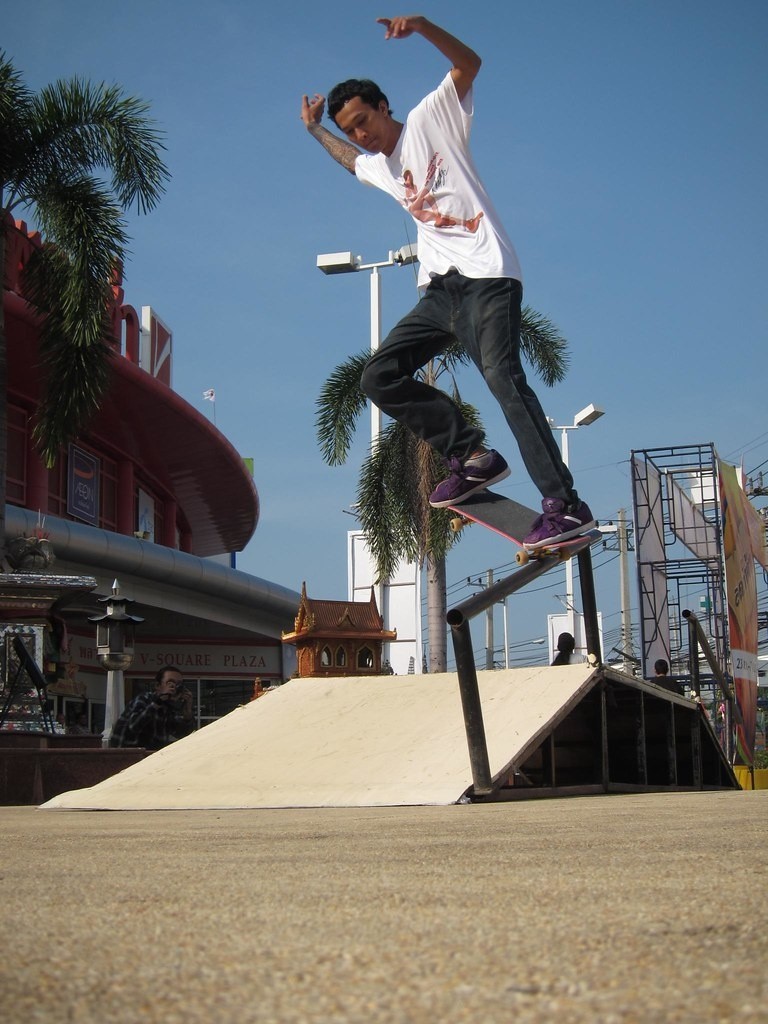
[170,683,184,697]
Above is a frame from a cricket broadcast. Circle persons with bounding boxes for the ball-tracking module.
[56,714,69,736]
[650,659,684,696]
[112,666,197,751]
[68,712,99,747]
[550,632,588,665]
[300,15,596,547]
[403,169,484,233]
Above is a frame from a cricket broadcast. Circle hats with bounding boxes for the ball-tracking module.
[556,633,575,651]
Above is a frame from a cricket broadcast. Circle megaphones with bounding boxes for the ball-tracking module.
[134,530,150,540]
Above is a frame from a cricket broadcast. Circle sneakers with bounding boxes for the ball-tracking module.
[522,497,598,551]
[426,449,511,509]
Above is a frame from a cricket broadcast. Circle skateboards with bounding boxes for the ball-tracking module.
[444,485,607,569]
